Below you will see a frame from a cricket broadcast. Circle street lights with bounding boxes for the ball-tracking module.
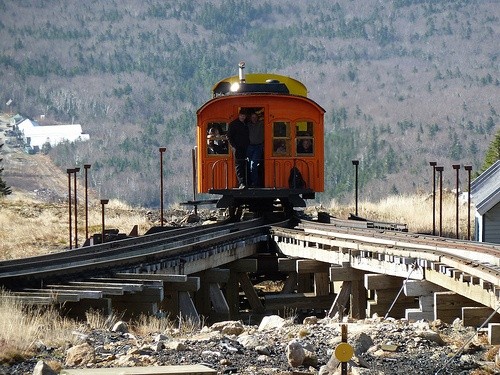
[83,164,91,240]
[158,147,167,228]
[100,199,110,243]
[435,166,444,237]
[67,167,81,249]
[452,165,460,239]
[429,161,437,236]
[352,160,359,216]
[464,165,472,240]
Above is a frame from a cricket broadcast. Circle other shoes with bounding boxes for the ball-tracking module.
[239,183,248,189]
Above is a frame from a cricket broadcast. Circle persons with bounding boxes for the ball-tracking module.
[206,122,228,154]
[247,112,265,188]
[274,138,313,154]
[227,110,250,189]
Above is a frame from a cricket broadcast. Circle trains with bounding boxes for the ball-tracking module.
[193,60,327,222]
